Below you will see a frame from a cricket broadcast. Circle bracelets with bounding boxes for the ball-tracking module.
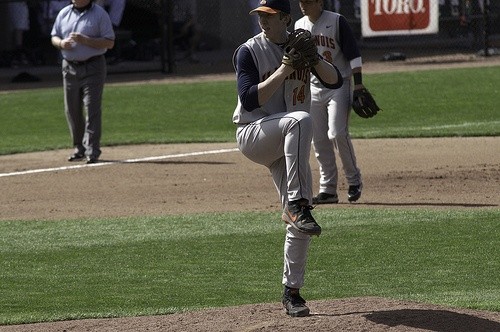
[353,72,362,84]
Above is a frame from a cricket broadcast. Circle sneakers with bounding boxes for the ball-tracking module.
[282,294,310,317]
[347,182,362,202]
[312,192,339,204]
[282,198,321,237]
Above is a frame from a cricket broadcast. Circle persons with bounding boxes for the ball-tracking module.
[0,0,72,84]
[93,0,203,76]
[51,0,115,165]
[232,0,343,318]
[294,0,380,203]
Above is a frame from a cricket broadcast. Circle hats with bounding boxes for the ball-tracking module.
[249,0,291,17]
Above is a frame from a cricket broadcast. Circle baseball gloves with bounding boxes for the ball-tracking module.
[350,85,380,119]
[281,27,318,71]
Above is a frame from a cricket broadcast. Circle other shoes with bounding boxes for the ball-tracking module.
[86,156,97,164]
[67,153,85,161]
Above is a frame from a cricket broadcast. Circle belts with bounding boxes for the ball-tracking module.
[67,55,100,64]
